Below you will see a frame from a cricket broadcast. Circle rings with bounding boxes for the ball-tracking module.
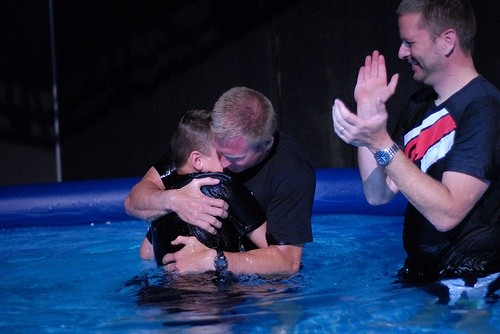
[338,129,344,134]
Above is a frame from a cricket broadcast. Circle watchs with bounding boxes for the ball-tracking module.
[212,247,228,276]
[374,142,399,169]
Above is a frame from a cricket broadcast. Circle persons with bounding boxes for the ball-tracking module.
[331,0,500,310]
[139,110,269,267]
[123,87,316,277]
